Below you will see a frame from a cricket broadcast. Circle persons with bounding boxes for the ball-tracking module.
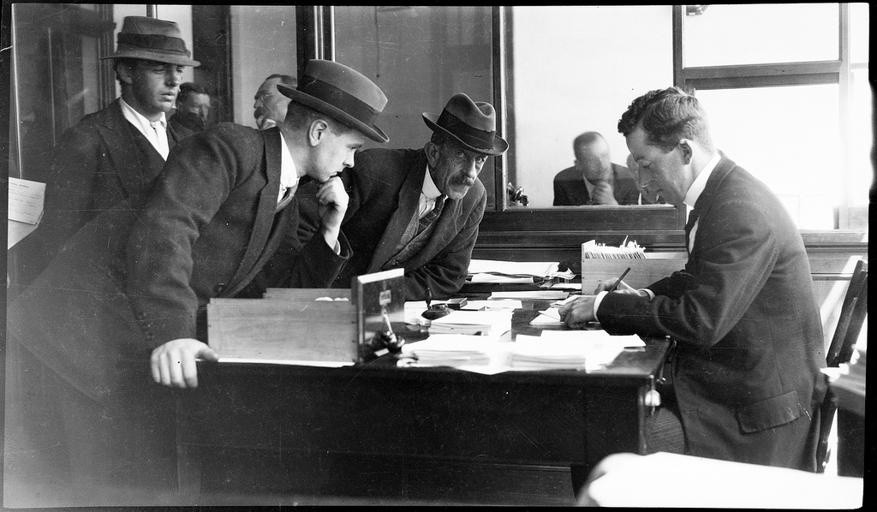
[250,72,300,132]
[0,57,391,510]
[313,94,511,306]
[548,131,642,209]
[554,82,832,476]
[34,16,208,257]
[174,80,212,131]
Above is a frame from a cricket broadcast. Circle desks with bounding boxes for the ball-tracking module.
[112,278,676,508]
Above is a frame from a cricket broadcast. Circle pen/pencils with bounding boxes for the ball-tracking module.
[609,267,631,293]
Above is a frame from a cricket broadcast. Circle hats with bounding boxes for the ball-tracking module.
[421,93,509,156]
[101,16,201,68]
[276,58,391,144]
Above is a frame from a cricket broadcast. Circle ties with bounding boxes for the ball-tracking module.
[419,194,446,236]
[684,210,698,255]
